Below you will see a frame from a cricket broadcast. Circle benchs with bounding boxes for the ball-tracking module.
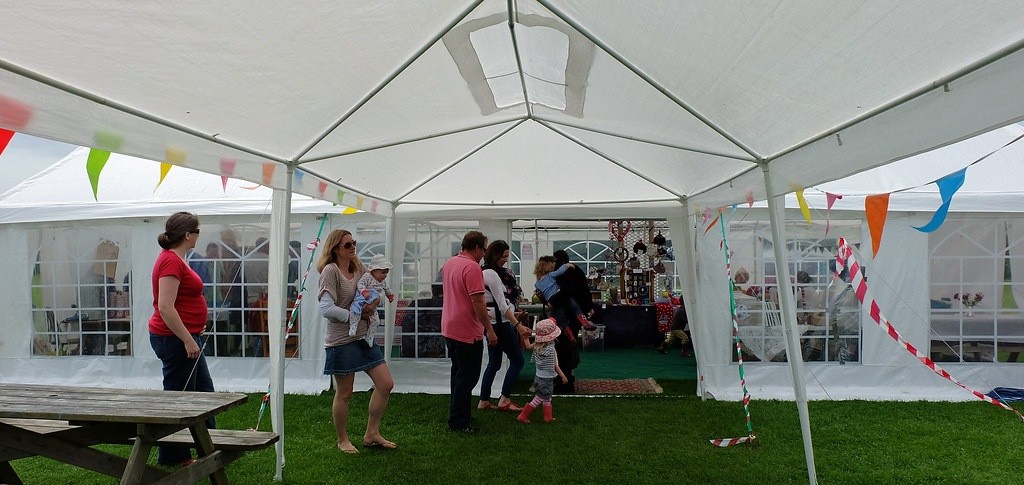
[929,345,1023,362]
[0,417,280,485]
[733,335,834,363]
[737,325,834,360]
[839,335,858,360]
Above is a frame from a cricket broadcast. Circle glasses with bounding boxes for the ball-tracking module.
[479,246,486,252]
[339,240,356,249]
[190,229,199,234]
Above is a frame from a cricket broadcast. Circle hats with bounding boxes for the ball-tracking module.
[535,319,561,342]
[368,254,394,271]
[290,241,301,248]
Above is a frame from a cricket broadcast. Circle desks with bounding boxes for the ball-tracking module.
[63,317,131,355]
[732,291,1024,362]
[0,383,247,485]
[594,305,659,347]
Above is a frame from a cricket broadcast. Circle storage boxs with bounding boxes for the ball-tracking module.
[581,324,606,353]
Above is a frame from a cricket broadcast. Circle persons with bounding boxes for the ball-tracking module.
[735,266,821,333]
[533,258,597,342]
[517,317,567,424]
[478,238,533,411]
[441,230,498,431]
[149,210,218,466]
[656,294,693,357]
[187,230,301,360]
[348,252,394,348]
[535,250,596,393]
[315,229,399,453]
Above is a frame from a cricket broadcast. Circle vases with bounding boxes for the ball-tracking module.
[966,305,977,317]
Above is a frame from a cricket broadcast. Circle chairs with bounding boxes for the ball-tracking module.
[46,311,80,356]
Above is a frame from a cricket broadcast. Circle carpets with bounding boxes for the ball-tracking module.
[530,377,664,394]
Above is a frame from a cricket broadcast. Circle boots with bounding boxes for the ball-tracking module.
[682,344,691,356]
[544,404,555,421]
[578,314,597,331]
[516,403,535,424]
[656,341,669,354]
[564,327,577,344]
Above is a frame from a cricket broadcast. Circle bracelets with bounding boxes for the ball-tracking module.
[515,322,520,328]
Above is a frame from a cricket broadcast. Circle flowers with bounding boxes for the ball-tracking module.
[952,291,982,306]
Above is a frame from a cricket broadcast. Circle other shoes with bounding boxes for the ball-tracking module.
[180,458,197,468]
[450,424,481,433]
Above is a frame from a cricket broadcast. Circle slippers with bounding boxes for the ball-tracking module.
[339,444,360,457]
[498,401,524,413]
[478,402,498,410]
[362,440,397,450]
